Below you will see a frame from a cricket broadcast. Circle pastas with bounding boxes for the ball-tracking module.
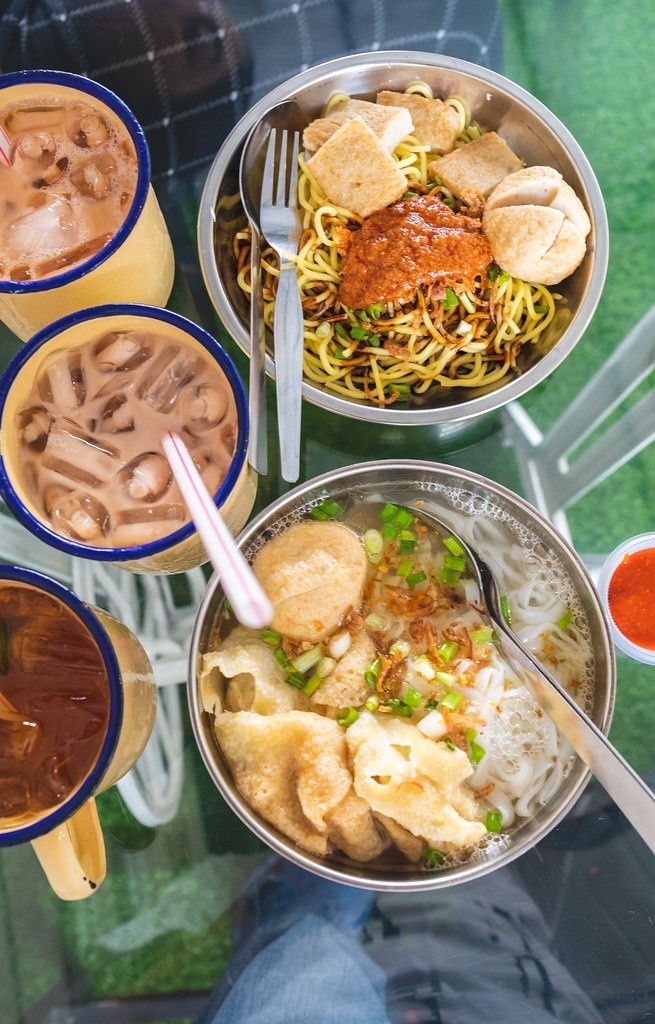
[232,83,562,408]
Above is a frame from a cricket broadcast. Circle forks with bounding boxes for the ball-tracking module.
[260,121,309,486]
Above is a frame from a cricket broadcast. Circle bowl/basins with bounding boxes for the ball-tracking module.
[195,47,609,426]
[601,527,655,670]
[185,449,624,895]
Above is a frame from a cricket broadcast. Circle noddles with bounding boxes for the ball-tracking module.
[198,494,593,866]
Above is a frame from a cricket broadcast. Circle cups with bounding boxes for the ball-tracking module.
[1,564,160,908]
[1,65,177,349]
[1,299,258,575]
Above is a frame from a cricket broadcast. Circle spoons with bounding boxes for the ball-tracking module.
[237,99,305,479]
[347,494,654,857]
